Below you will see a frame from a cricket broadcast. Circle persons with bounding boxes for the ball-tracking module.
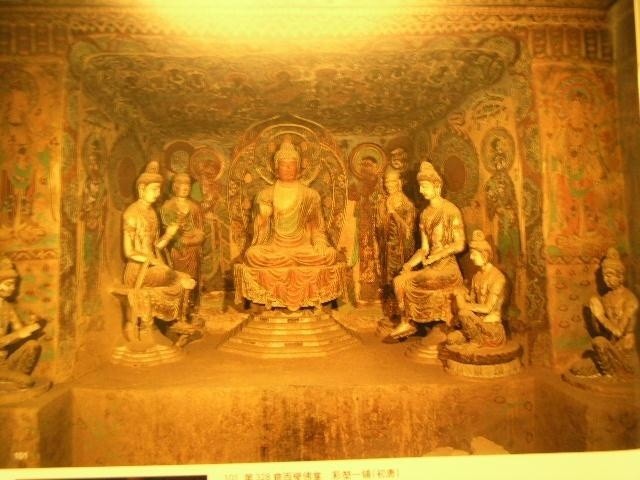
[453,232,509,348]
[544,82,609,237]
[1,82,49,239]
[372,160,418,329]
[1,258,43,389]
[383,160,468,340]
[117,151,174,355]
[570,253,639,387]
[162,168,207,322]
[64,166,113,311]
[235,138,339,267]
